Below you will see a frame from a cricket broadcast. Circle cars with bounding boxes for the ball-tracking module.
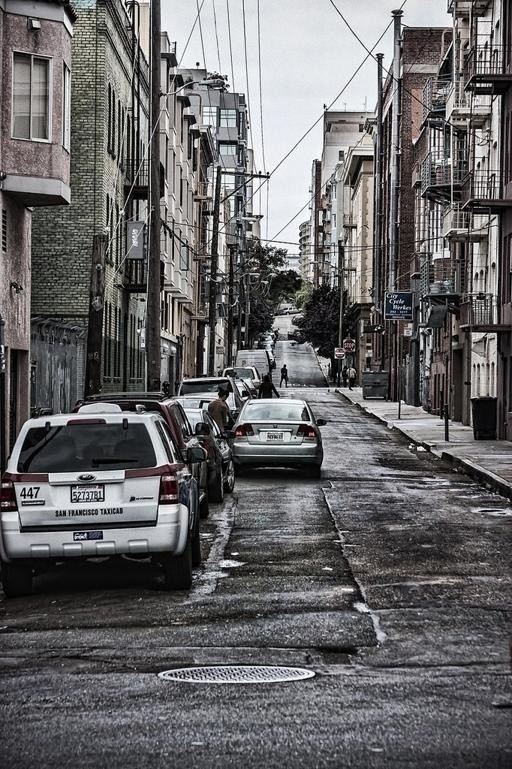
[221,330,277,404]
[160,393,235,446]
[182,406,238,503]
[232,398,328,475]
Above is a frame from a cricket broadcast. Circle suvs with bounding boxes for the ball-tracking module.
[0,406,209,600]
[178,373,245,412]
[70,388,208,521]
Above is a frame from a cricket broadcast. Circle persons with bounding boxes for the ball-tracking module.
[343,364,356,391]
[280,364,288,388]
[274,328,280,340]
[258,375,280,398]
[208,390,229,432]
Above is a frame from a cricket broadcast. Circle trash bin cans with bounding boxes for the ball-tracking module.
[470,395,497,440]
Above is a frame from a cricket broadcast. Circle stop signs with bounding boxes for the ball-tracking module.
[334,347,346,360]
